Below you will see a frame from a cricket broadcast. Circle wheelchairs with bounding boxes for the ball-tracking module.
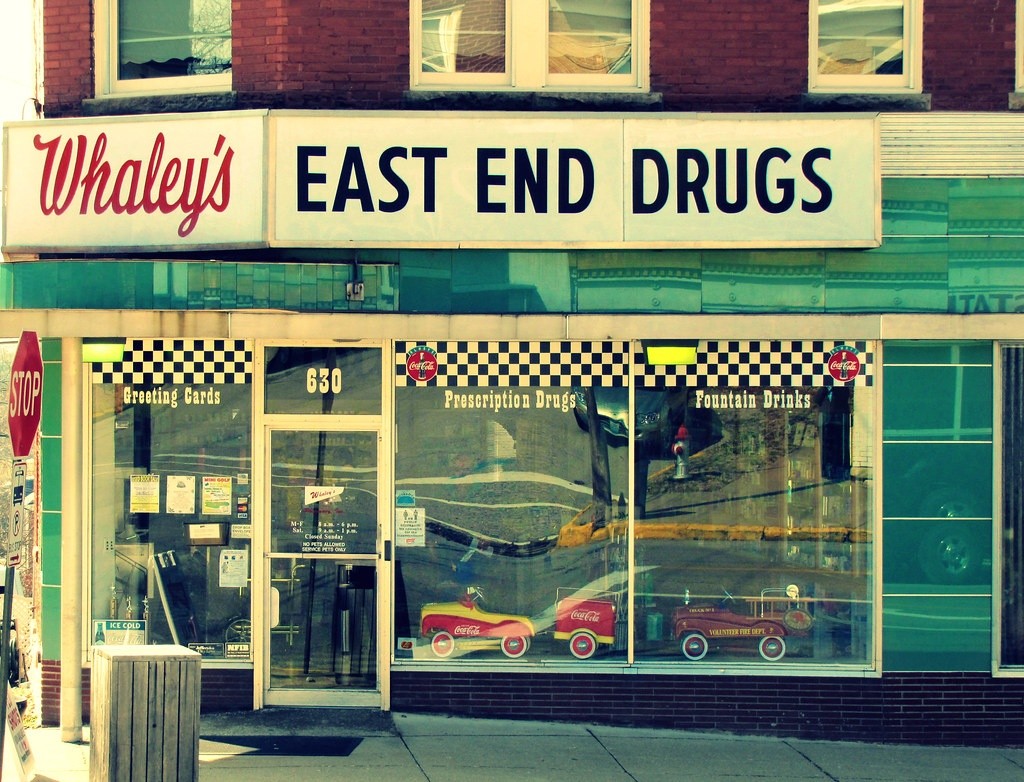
[222,564,315,648]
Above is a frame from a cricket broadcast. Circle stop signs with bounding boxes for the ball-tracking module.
[9,331,44,457]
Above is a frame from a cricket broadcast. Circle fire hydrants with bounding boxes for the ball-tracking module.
[670,423,693,479]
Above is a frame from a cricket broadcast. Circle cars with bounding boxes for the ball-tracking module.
[894,403,993,518]
[571,386,691,443]
[605,543,872,648]
